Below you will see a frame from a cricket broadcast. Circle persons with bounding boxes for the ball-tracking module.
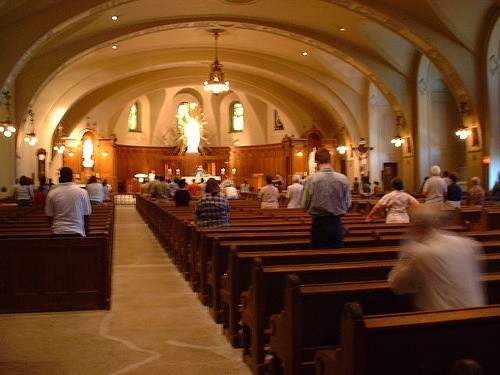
[17,176,35,207]
[301,148,352,250]
[241,181,249,193]
[103,179,112,202]
[150,175,205,198]
[87,176,104,205]
[35,177,54,209]
[258,175,280,209]
[45,167,92,237]
[207,176,239,199]
[422,165,463,209]
[467,177,484,205]
[174,180,190,207]
[353,177,382,200]
[195,181,230,229]
[286,174,304,209]
[366,178,419,223]
[388,208,486,311]
[272,173,283,192]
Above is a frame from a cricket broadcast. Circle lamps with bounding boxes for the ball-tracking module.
[0,90,37,146]
[203,29,229,94]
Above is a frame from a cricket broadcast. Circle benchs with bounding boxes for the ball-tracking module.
[0,193,116,313]
[135,191,500,375]
